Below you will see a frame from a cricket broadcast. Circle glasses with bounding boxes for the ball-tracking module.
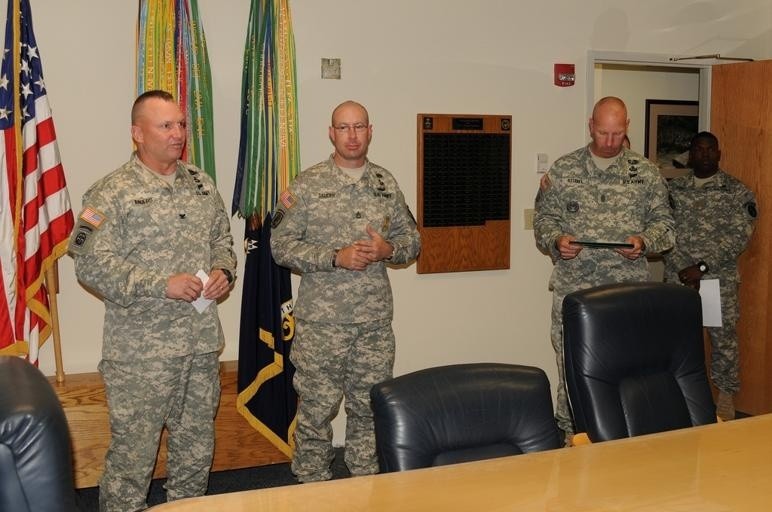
[332,123,368,135]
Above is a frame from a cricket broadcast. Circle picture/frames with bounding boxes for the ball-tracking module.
[642,97,698,178]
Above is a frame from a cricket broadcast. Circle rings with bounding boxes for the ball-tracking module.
[683,276,686,280]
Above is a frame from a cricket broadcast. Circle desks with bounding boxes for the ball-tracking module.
[143,413,770,511]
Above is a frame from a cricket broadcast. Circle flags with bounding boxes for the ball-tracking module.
[1,0,75,370]
[234,211,318,459]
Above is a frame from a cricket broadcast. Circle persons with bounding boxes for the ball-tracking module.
[270,102,421,484]
[532,96,675,448]
[620,134,631,150]
[659,132,759,422]
[67,90,238,512]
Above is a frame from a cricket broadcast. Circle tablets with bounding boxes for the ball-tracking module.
[569,241,634,249]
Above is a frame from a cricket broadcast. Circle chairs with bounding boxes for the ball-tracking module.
[562,280,728,446]
[0,352,78,512]
[366,361,560,474]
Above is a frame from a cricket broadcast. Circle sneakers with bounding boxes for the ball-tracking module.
[716,391,736,423]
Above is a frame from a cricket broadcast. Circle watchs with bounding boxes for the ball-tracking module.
[699,262,709,274]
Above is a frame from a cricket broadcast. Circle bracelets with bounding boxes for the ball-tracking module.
[386,242,395,260]
[332,248,339,267]
[222,268,233,283]
